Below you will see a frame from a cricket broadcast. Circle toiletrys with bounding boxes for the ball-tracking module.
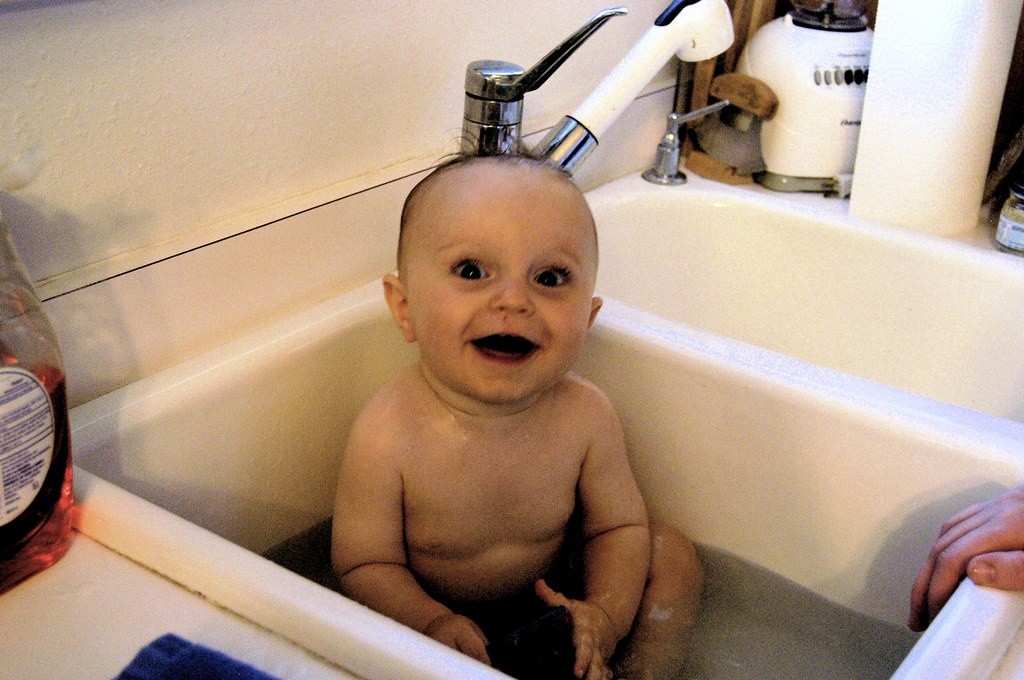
[0,213,75,595]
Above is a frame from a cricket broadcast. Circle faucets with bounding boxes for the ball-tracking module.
[527,0,737,176]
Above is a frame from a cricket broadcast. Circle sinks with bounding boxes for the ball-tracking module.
[76,299,1019,680]
[572,192,1024,424]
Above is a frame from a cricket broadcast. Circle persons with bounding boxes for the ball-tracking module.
[332,155,705,680]
[909,485,1024,631]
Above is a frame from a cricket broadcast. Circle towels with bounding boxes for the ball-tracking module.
[109,632,278,680]
